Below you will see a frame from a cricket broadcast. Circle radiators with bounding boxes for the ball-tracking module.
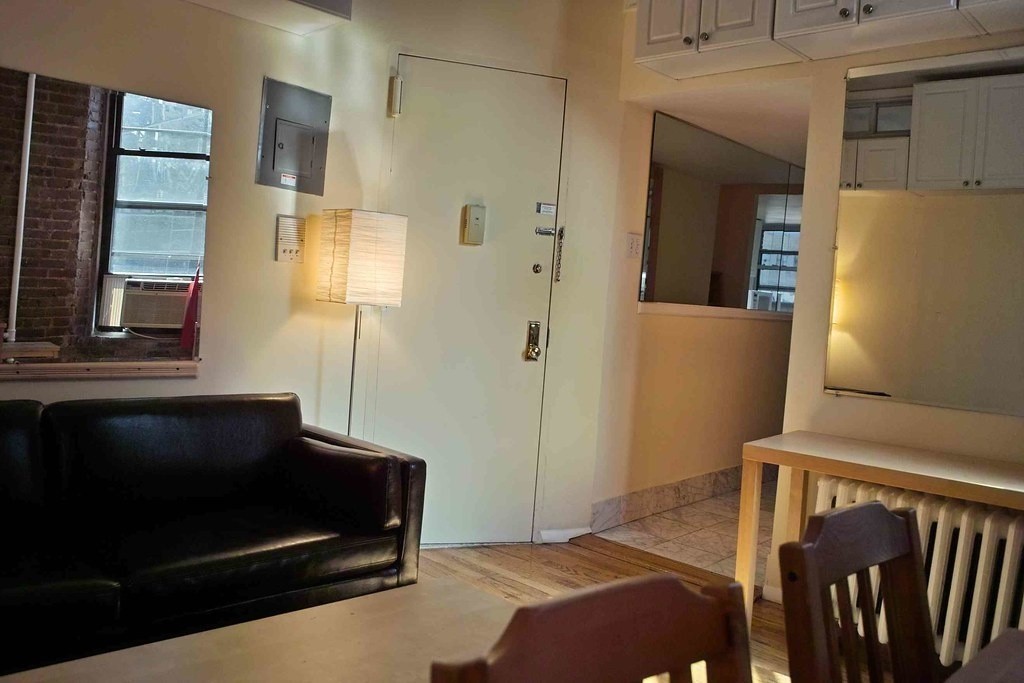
[99,273,126,326]
[814,473,1024,668]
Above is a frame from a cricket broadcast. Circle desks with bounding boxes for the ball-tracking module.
[943,627,1024,683]
[733,430,1024,645]
[0,342,60,364]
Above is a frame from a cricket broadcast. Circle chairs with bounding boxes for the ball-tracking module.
[430,572,752,683]
[778,499,944,683]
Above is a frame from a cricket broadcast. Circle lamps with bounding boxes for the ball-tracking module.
[312,208,409,308]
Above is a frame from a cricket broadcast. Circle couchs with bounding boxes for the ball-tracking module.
[0,392,426,676]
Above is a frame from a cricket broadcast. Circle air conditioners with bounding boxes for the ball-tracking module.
[121,279,202,329]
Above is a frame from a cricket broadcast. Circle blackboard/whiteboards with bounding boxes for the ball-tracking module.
[823,188,1024,418]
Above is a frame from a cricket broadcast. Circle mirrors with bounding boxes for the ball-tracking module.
[0,67,214,379]
[775,163,805,321]
[637,110,790,320]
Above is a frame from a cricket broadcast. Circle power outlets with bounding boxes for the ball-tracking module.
[626,232,643,258]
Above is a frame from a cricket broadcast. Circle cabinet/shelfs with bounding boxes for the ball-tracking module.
[773,0,982,61]
[634,0,809,80]
[958,0,1024,36]
[839,137,909,191]
[844,101,910,137]
[756,223,800,315]
[906,72,1024,196]
